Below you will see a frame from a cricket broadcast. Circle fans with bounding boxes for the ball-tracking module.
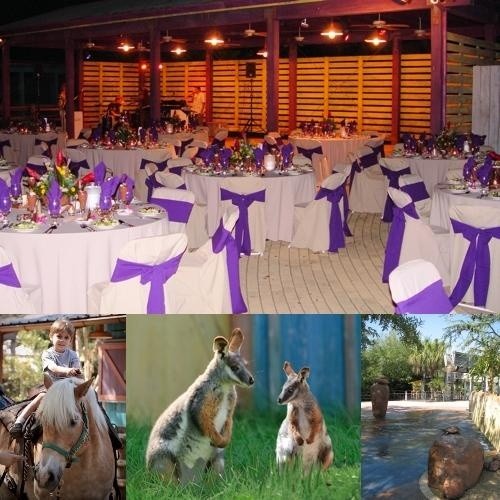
[352,13,409,30]
[83,38,107,50]
[225,24,267,37]
[399,17,431,40]
[160,30,187,43]
[133,40,150,51]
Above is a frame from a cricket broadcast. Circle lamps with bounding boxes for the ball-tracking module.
[117,39,134,51]
[256,39,268,57]
[364,31,387,46]
[320,22,343,39]
[204,29,224,46]
[89,324,112,339]
[170,46,187,55]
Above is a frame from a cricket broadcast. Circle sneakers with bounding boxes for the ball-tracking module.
[9,423,23,439]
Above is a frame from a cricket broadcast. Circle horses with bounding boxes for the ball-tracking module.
[0,372,118,500]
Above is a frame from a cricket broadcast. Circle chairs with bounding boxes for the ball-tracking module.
[0,127,457,314]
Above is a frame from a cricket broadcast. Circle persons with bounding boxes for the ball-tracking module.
[106,97,123,124]
[9,319,84,437]
[188,87,206,127]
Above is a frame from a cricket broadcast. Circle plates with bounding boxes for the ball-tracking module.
[447,178,500,200]
[184,164,313,175]
[10,206,165,232]
